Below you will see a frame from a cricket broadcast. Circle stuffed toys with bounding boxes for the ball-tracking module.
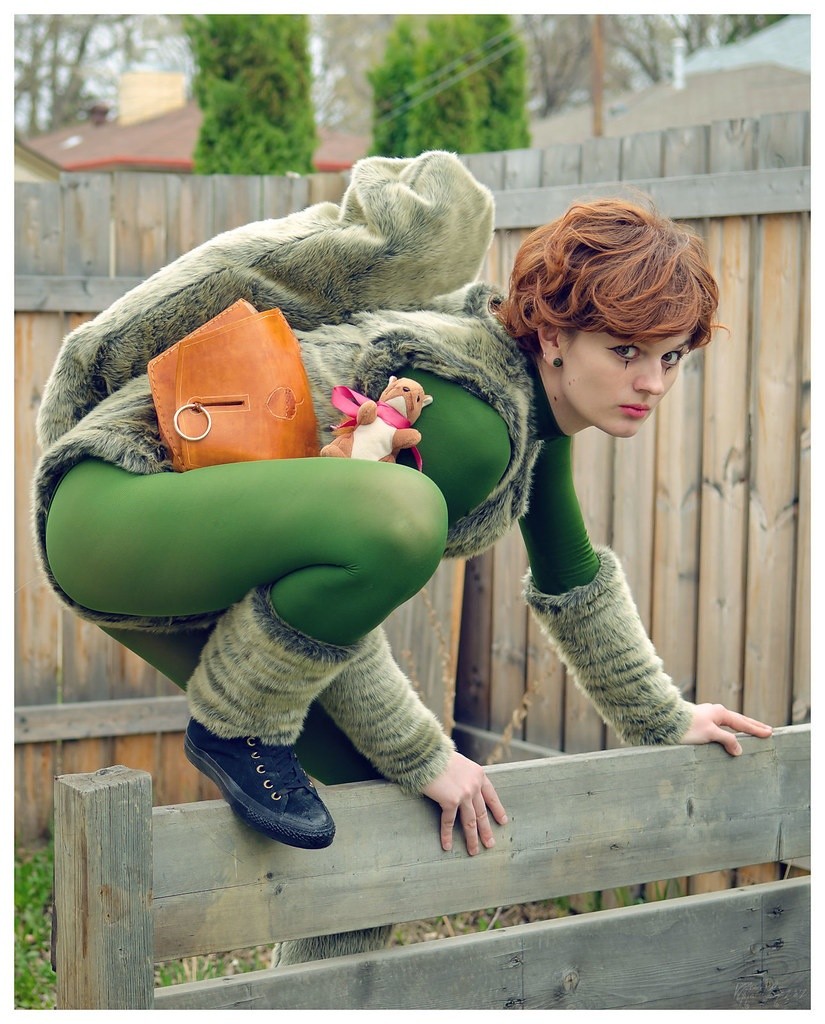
[319,373,432,464]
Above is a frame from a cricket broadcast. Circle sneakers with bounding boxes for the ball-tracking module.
[185,717,336,851]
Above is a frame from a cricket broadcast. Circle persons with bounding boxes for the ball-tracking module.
[34,152,772,969]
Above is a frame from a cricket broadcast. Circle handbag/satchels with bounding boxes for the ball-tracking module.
[145,297,318,476]
